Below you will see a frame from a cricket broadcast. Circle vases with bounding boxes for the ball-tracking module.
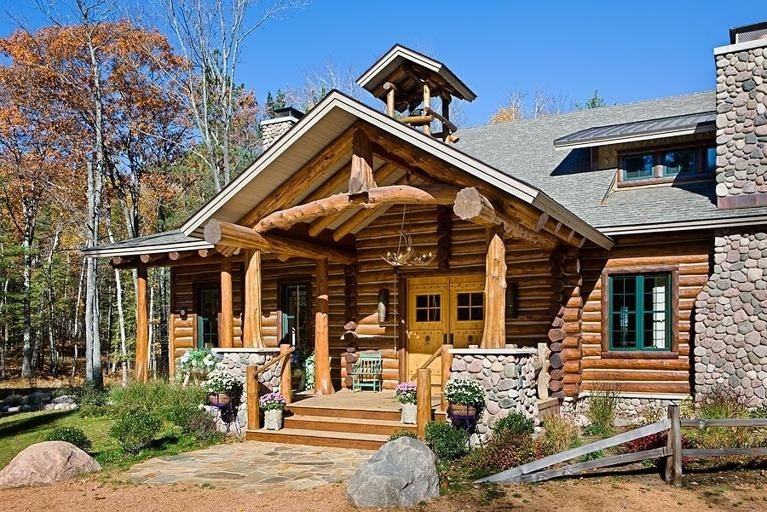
[448,401,478,435]
[263,409,284,430]
[399,403,417,425]
[208,392,231,408]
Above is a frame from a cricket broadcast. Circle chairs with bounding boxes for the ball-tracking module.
[349,351,384,394]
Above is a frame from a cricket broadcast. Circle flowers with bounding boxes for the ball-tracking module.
[444,378,485,410]
[198,372,243,397]
[394,383,418,406]
[181,347,216,373]
[258,392,287,411]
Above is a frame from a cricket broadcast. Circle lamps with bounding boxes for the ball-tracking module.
[380,204,436,268]
